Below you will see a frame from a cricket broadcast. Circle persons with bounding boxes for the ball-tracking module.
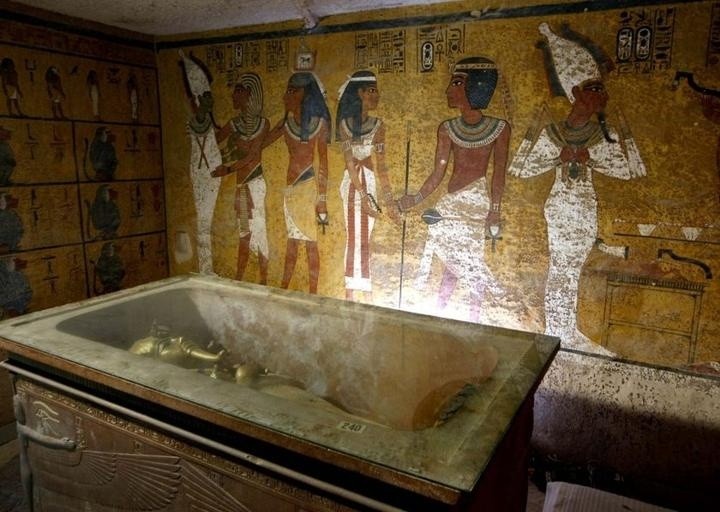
[234,72,334,296]
[333,72,402,306]
[86,69,104,120]
[45,64,68,119]
[185,72,273,287]
[126,75,140,122]
[507,75,645,360]
[398,55,511,324]
[128,324,388,429]
[0,57,26,117]
[183,59,229,276]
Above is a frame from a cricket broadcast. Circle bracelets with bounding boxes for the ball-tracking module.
[358,188,368,199]
[227,165,231,174]
[385,191,393,202]
[490,203,502,214]
[413,192,424,204]
[319,194,329,202]
